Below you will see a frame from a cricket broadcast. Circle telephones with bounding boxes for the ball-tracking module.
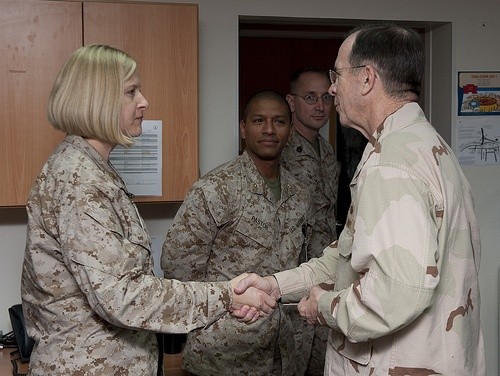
[8,304,37,364]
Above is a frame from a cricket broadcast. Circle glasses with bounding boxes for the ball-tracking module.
[290,93,333,105]
[329,65,378,83]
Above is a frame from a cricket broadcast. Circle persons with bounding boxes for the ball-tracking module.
[21,43,277,376]
[161,91,316,376]
[279,65,341,376]
[230,21,486,376]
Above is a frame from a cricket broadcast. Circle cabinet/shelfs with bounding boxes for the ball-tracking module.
[0,0,201,210]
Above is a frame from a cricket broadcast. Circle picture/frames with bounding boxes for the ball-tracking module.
[457,71,500,116]
